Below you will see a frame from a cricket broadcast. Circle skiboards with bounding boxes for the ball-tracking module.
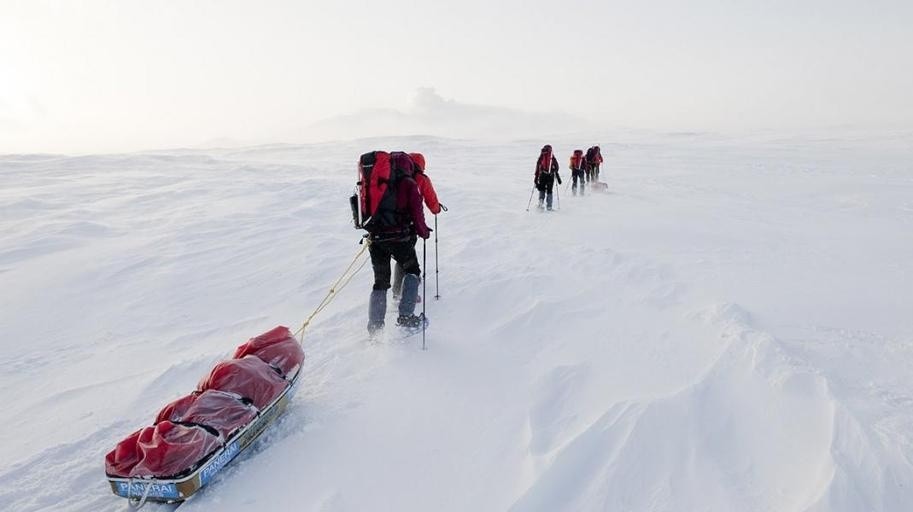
[364,313,429,342]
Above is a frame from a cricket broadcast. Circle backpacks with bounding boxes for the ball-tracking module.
[540,148,552,173]
[574,153,582,169]
[356,151,412,240]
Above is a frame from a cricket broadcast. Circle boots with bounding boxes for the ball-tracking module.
[396,274,425,327]
[368,289,385,334]
[547,194,552,209]
[539,192,544,205]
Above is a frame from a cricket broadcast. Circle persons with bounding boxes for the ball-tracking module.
[568,146,604,197]
[534,144,561,211]
[392,153,449,303]
[365,151,433,339]
